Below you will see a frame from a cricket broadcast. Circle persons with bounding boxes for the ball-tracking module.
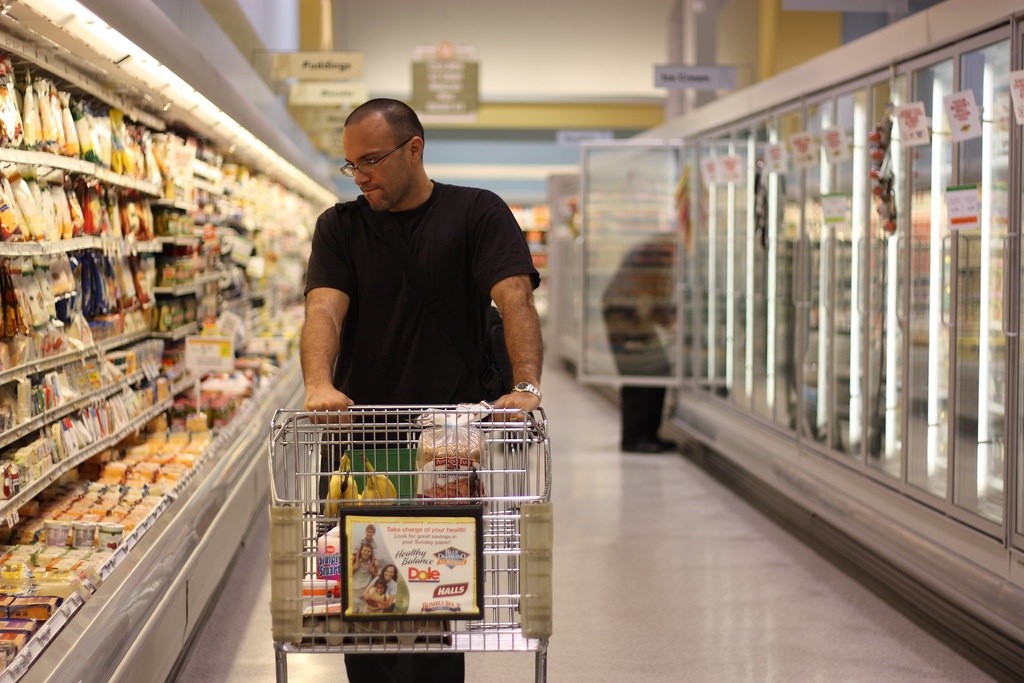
[364,564,398,613]
[603,229,679,454]
[351,543,381,614]
[291,98,547,683]
[363,580,391,614]
[355,523,377,566]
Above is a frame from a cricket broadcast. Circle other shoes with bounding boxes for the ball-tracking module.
[623,438,663,451]
[651,435,676,449]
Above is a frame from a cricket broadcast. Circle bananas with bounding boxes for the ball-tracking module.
[324,449,397,519]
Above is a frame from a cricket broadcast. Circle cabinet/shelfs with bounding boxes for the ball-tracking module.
[0,32,337,683]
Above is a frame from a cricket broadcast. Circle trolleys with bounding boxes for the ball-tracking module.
[266,400,555,683]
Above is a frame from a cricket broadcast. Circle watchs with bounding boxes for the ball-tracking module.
[512,381,543,401]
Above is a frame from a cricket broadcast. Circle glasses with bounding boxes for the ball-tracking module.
[340,138,411,177]
